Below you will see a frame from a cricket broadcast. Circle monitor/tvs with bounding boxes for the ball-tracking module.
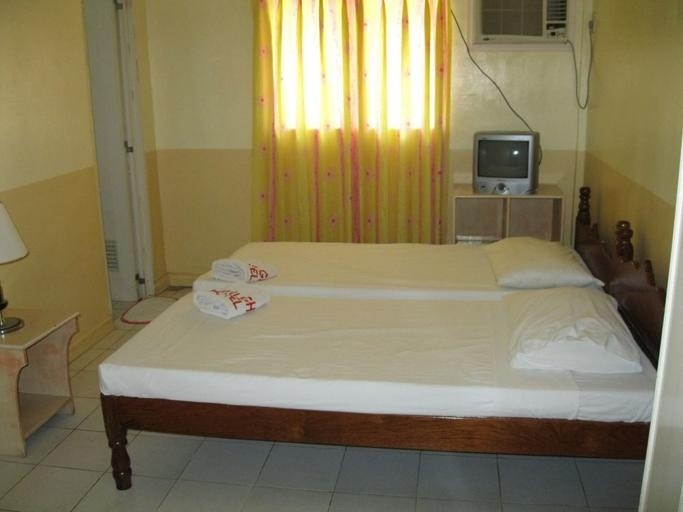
[473,131,540,194]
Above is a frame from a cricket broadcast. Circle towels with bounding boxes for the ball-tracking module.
[191,256,278,320]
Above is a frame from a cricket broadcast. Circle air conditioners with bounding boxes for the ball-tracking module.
[470,0,573,46]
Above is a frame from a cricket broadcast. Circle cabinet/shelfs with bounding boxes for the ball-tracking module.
[0,307,82,458]
[452,182,564,246]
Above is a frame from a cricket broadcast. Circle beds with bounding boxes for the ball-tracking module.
[97,186,670,498]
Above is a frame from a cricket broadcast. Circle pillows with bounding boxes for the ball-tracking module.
[480,237,643,374]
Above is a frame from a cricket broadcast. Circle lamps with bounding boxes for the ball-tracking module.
[0,203,30,334]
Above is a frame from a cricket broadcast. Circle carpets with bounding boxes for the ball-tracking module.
[113,294,177,331]
